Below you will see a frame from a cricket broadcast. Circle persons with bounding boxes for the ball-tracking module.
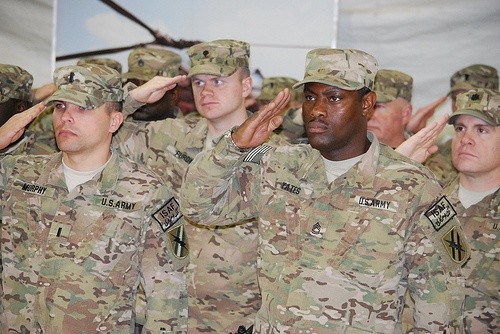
[0,47,200,157]
[0,63,189,334]
[179,46,470,334]
[366,64,500,188]
[245,76,310,147]
[105,39,295,334]
[392,89,500,334]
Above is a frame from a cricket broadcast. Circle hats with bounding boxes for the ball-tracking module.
[291,49,378,92]
[187,40,251,77]
[77,58,122,73]
[447,63,499,96]
[0,64,33,103]
[44,64,126,110]
[446,88,500,127]
[256,77,304,103]
[120,48,182,81]
[374,69,414,102]
